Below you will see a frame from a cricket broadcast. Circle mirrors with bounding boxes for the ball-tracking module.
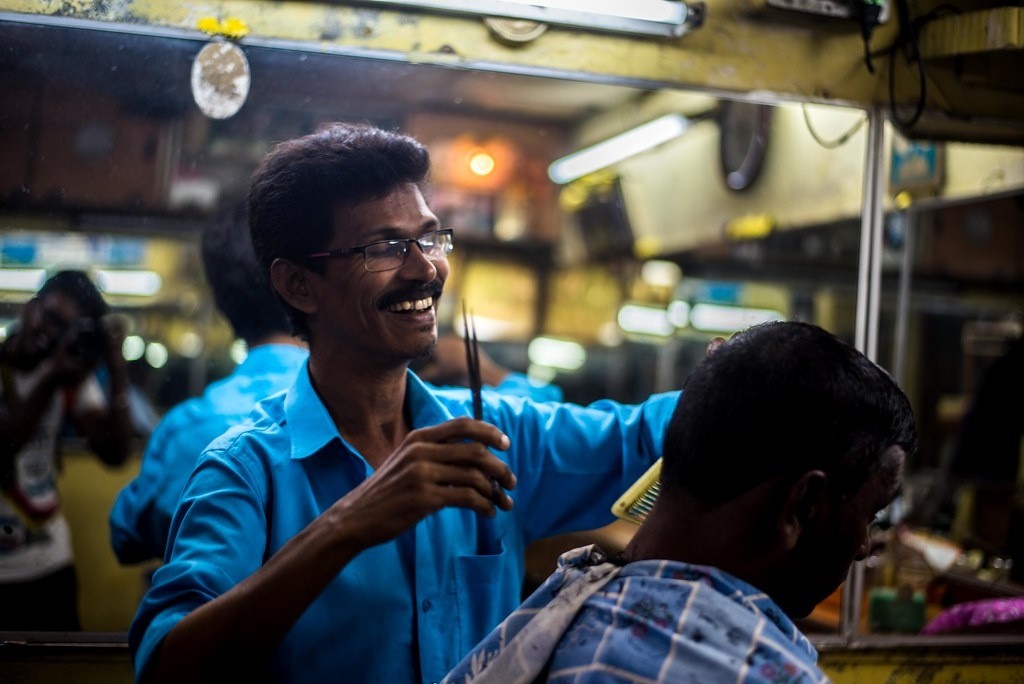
[1,11,1024,668]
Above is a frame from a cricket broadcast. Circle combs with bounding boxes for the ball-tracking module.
[610,457,663,526]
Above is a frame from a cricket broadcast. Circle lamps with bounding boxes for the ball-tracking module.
[389,0,707,38]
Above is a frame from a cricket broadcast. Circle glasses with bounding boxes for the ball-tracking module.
[292,229,453,272]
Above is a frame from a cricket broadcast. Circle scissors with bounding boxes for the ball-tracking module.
[461,299,483,420]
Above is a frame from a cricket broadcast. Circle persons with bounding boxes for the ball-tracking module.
[912,333,1024,573]
[126,125,723,667]
[0,270,135,637]
[108,174,562,566]
[436,320,919,684]
